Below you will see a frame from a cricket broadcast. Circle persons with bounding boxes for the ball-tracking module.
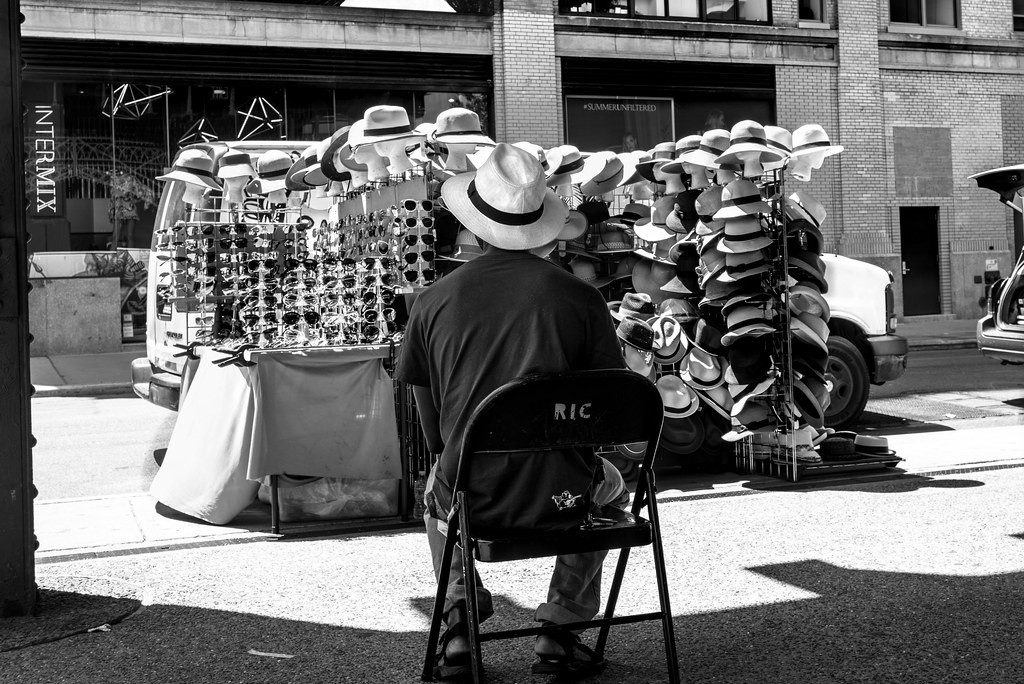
[393,144,629,684]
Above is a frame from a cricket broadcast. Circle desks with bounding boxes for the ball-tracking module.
[183,343,412,539]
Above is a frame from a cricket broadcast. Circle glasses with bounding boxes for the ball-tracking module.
[152,189,438,345]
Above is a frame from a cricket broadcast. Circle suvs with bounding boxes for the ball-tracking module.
[127,133,907,437]
[975,158,1024,371]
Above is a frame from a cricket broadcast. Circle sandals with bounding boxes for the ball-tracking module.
[431,624,472,677]
[532,628,608,680]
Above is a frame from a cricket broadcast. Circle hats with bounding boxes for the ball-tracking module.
[442,144,566,250]
[155,108,895,468]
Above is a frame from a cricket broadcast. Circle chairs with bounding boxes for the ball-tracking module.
[419,365,681,684]
